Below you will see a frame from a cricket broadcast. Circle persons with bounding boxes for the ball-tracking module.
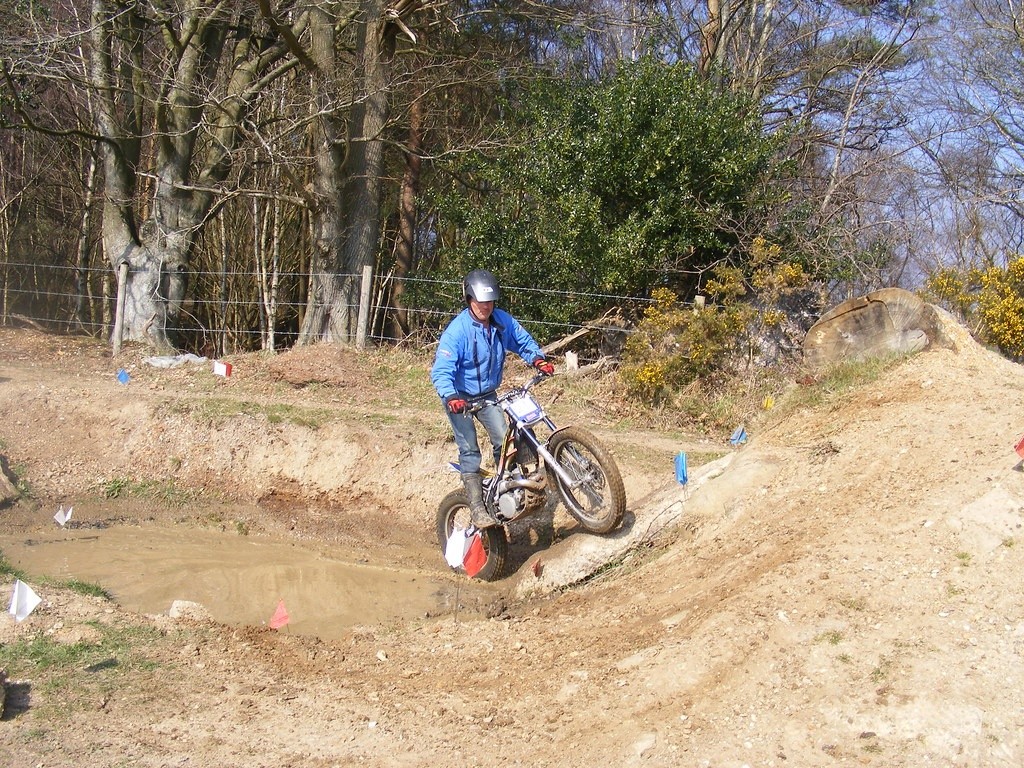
[430,270,555,527]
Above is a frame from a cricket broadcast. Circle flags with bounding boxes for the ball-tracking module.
[117,370,128,384]
[445,526,486,578]
[270,601,289,629]
[214,361,233,377]
[763,397,773,410]
[54,505,73,526]
[730,425,747,446]
[675,451,688,485]
[9,580,42,622]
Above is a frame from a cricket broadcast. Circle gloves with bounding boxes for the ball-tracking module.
[446,398,468,414]
[535,359,555,376]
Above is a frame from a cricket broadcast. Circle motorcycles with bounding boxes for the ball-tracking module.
[435,370,626,583]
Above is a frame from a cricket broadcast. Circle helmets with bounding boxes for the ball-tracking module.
[464,270,499,308]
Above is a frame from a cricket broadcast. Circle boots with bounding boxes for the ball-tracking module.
[462,473,496,528]
